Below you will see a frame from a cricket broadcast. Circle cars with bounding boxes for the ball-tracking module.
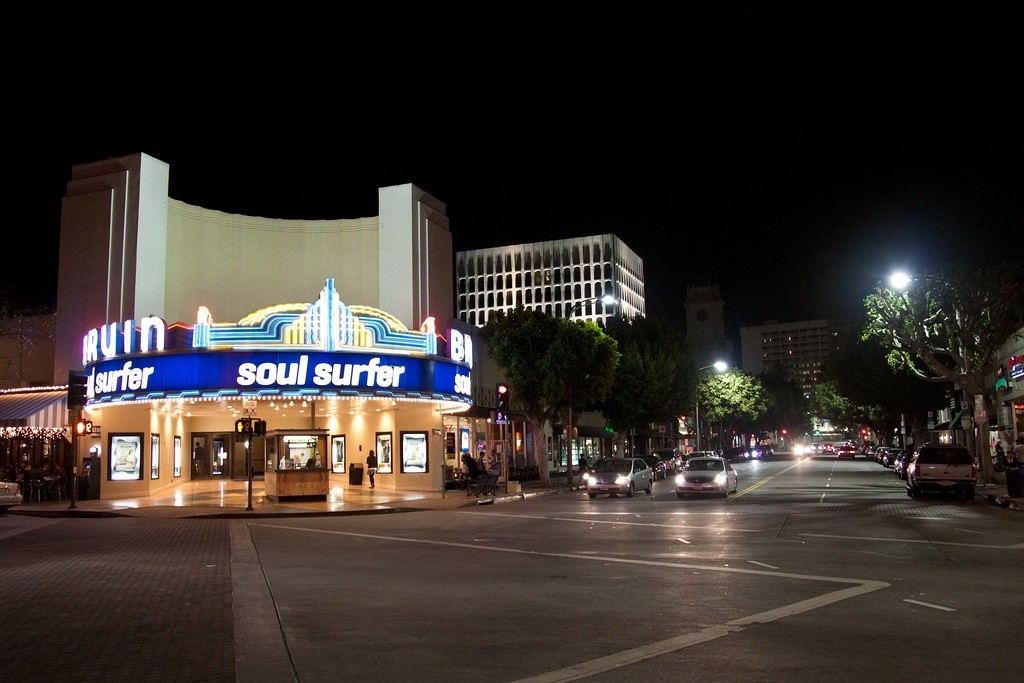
[810,442,857,458]
[0,471,22,514]
[676,456,738,499]
[861,440,912,479]
[635,447,718,480]
[725,445,778,462]
[587,458,653,498]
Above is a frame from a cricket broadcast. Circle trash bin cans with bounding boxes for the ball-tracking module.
[1002,462,1024,498]
[349,463,363,485]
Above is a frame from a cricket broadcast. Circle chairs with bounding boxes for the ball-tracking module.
[467,473,500,497]
[17,475,71,503]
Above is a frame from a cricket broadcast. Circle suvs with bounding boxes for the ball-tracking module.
[906,441,978,499]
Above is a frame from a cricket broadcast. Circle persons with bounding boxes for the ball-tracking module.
[300,453,307,465]
[0,460,70,504]
[455,444,500,493]
[194,442,207,479]
[982,422,1024,478]
[366,449,378,489]
[576,453,592,490]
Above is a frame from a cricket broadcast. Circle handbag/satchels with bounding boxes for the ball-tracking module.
[366,470,374,475]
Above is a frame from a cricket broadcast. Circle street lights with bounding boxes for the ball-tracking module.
[695,362,729,449]
[552,296,618,484]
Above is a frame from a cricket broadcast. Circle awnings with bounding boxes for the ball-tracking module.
[553,423,615,440]
[929,419,950,433]
[0,391,70,429]
[947,410,966,430]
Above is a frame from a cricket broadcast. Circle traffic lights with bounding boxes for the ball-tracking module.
[67,368,90,411]
[497,382,511,420]
[235,417,266,438]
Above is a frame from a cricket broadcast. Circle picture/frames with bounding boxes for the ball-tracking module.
[107,430,145,482]
[330,433,347,475]
[399,429,430,474]
[374,431,394,475]
[150,432,160,481]
[173,433,181,478]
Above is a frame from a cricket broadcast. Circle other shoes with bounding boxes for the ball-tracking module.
[369,484,374,488]
